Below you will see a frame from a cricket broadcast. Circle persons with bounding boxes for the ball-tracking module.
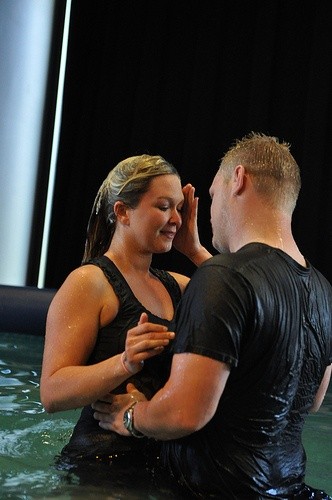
[90,132,331,500]
[38,154,213,484]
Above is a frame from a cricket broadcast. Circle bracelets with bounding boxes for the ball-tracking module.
[120,352,133,375]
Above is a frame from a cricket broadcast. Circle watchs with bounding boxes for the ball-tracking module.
[123,401,144,438]
